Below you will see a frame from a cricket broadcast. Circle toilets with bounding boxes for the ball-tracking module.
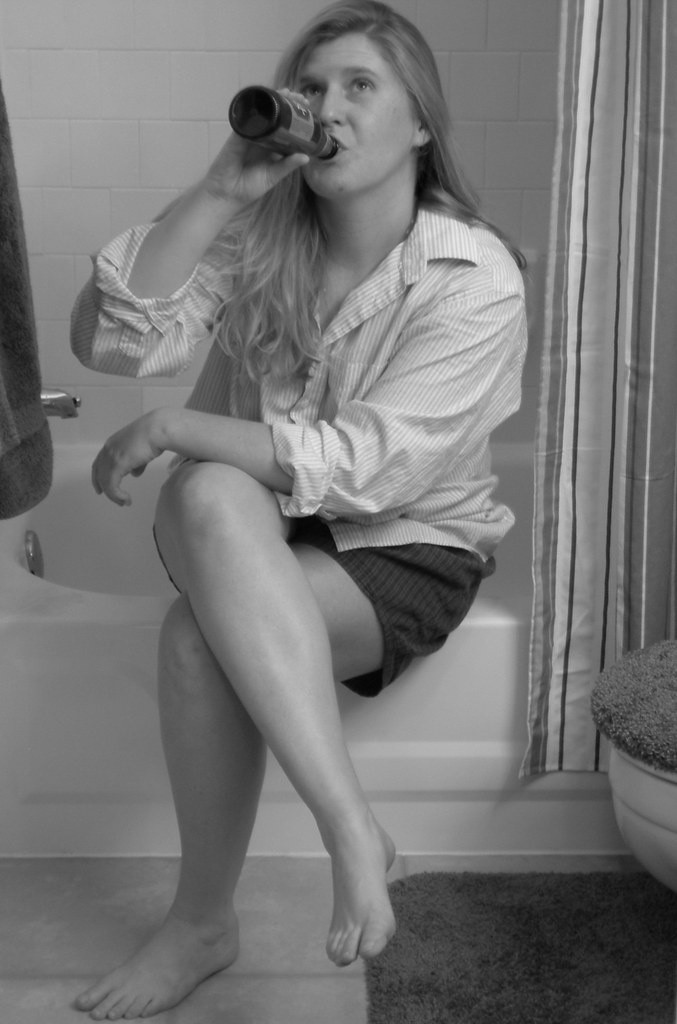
[609,739,676,894]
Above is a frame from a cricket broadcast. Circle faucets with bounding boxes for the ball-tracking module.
[41,388,82,419]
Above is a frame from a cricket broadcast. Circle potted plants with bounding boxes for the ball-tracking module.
[590,642,677,896]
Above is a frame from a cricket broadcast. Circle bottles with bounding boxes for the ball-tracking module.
[229,85,338,159]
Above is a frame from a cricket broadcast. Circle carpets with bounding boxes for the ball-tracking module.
[368,871,677,1024]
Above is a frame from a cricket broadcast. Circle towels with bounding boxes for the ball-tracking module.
[1,81,53,520]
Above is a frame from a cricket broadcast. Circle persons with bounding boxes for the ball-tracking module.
[68,1,526,1020]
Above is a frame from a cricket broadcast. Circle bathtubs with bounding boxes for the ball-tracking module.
[1,442,652,873]
